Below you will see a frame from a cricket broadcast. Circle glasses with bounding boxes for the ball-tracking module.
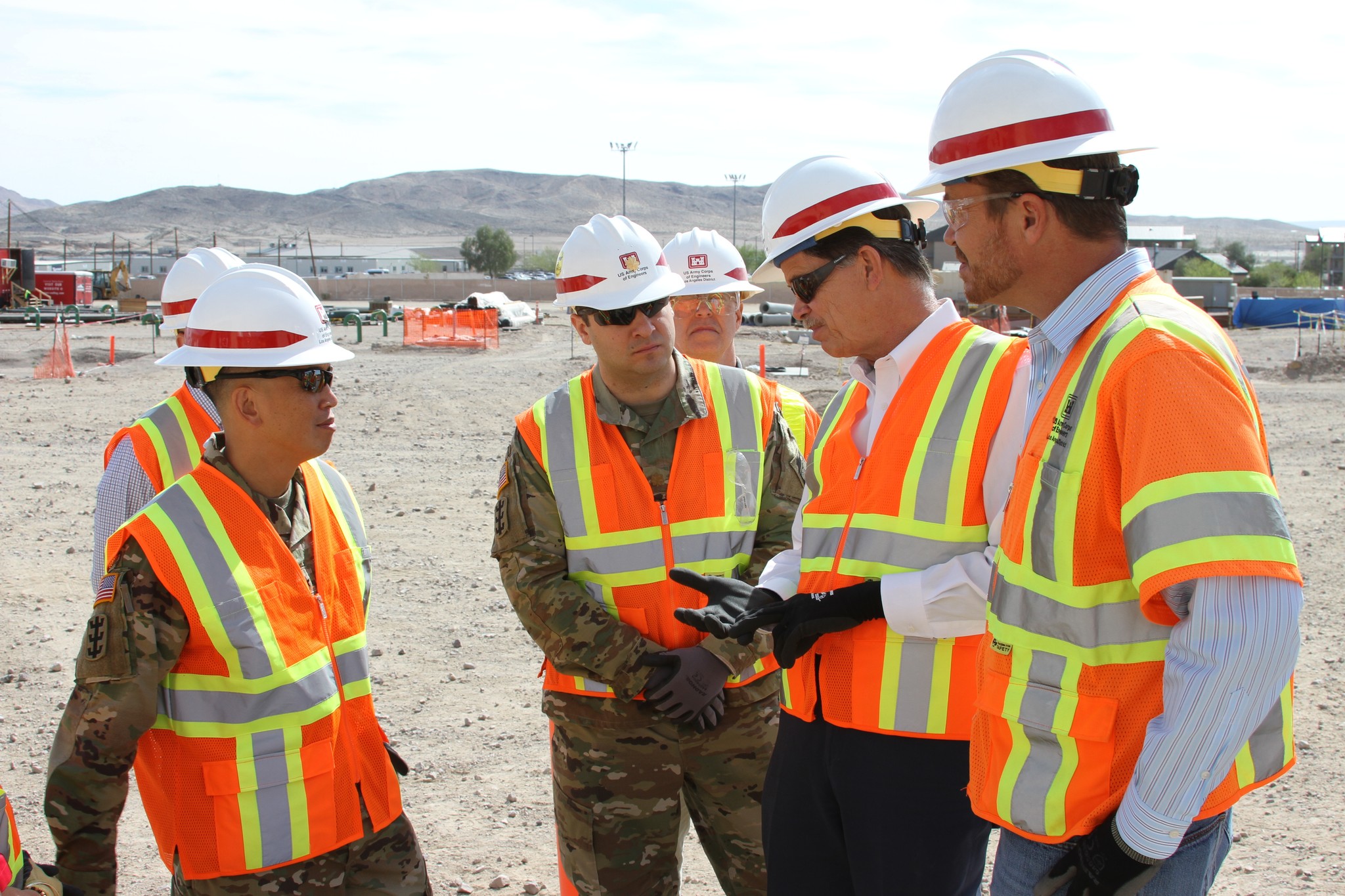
[670,291,741,317]
[215,369,334,392]
[790,254,848,303]
[942,191,1025,231]
[576,296,670,326]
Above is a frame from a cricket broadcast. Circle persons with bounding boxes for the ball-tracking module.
[659,225,826,464]
[91,245,247,595]
[486,212,807,896]
[43,263,432,896]
[665,155,1034,896]
[0,786,66,896]
[926,44,1316,896]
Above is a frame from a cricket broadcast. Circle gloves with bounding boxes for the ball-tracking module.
[729,579,886,670]
[668,567,783,646]
[644,663,727,733]
[638,645,730,725]
[1031,812,1165,896]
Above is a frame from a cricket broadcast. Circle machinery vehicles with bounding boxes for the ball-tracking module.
[77,260,132,301]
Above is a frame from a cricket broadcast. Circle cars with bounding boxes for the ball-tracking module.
[495,272,555,280]
[129,274,156,280]
[334,272,356,279]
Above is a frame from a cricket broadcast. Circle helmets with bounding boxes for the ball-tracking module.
[153,263,355,368]
[551,214,685,311]
[750,154,941,283]
[159,247,246,329]
[662,227,765,301]
[906,49,1160,198]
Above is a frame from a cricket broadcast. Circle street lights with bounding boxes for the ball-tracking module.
[725,173,746,249]
[609,140,638,218]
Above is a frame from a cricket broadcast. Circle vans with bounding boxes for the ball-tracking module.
[366,268,390,276]
